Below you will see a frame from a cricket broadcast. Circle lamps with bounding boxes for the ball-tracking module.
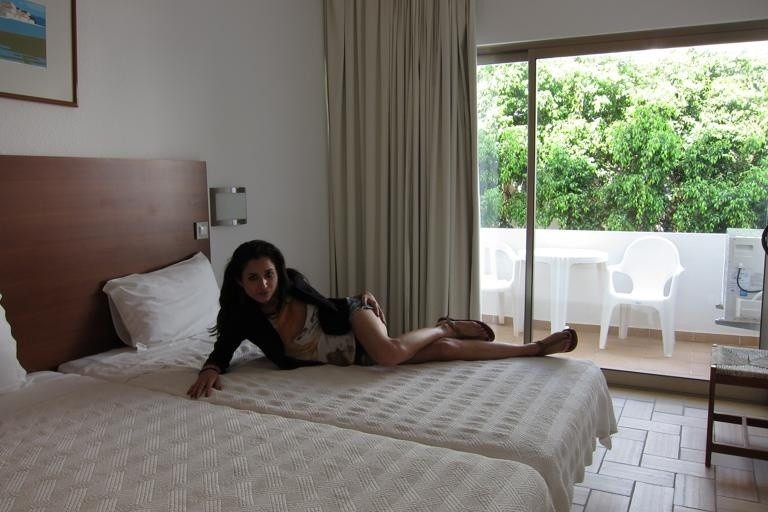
[209,187,247,226]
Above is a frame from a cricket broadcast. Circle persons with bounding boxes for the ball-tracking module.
[187,240,579,399]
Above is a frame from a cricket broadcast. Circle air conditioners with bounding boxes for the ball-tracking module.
[723,228,765,325]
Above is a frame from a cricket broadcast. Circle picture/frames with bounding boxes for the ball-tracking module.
[0,0,79,108]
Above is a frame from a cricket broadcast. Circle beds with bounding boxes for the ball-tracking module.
[59,330,620,512]
[0,370,558,512]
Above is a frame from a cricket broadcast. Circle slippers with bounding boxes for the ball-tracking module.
[435,317,496,341]
[532,328,579,355]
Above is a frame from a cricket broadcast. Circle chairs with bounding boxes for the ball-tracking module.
[478,236,520,338]
[599,235,686,358]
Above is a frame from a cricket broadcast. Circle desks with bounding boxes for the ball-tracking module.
[519,247,610,335]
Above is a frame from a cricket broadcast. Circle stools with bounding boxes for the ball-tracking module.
[705,343,768,470]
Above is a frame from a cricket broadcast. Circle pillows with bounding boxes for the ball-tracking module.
[102,250,221,354]
[0,294,28,395]
